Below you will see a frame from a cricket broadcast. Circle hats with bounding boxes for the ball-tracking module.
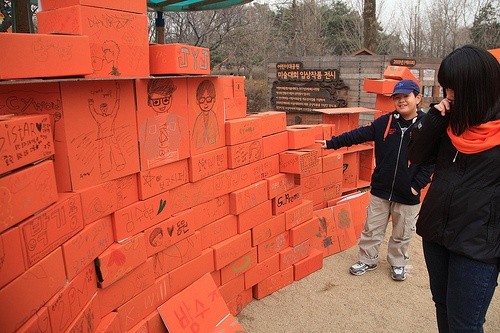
[390,80,420,97]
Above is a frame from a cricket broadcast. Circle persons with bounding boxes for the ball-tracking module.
[410,44,500,332]
[312,79,436,280]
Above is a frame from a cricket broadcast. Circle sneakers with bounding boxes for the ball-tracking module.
[390,266,406,281]
[349,261,377,276]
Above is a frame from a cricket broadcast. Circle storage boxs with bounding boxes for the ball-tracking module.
[0,0,422,333]
